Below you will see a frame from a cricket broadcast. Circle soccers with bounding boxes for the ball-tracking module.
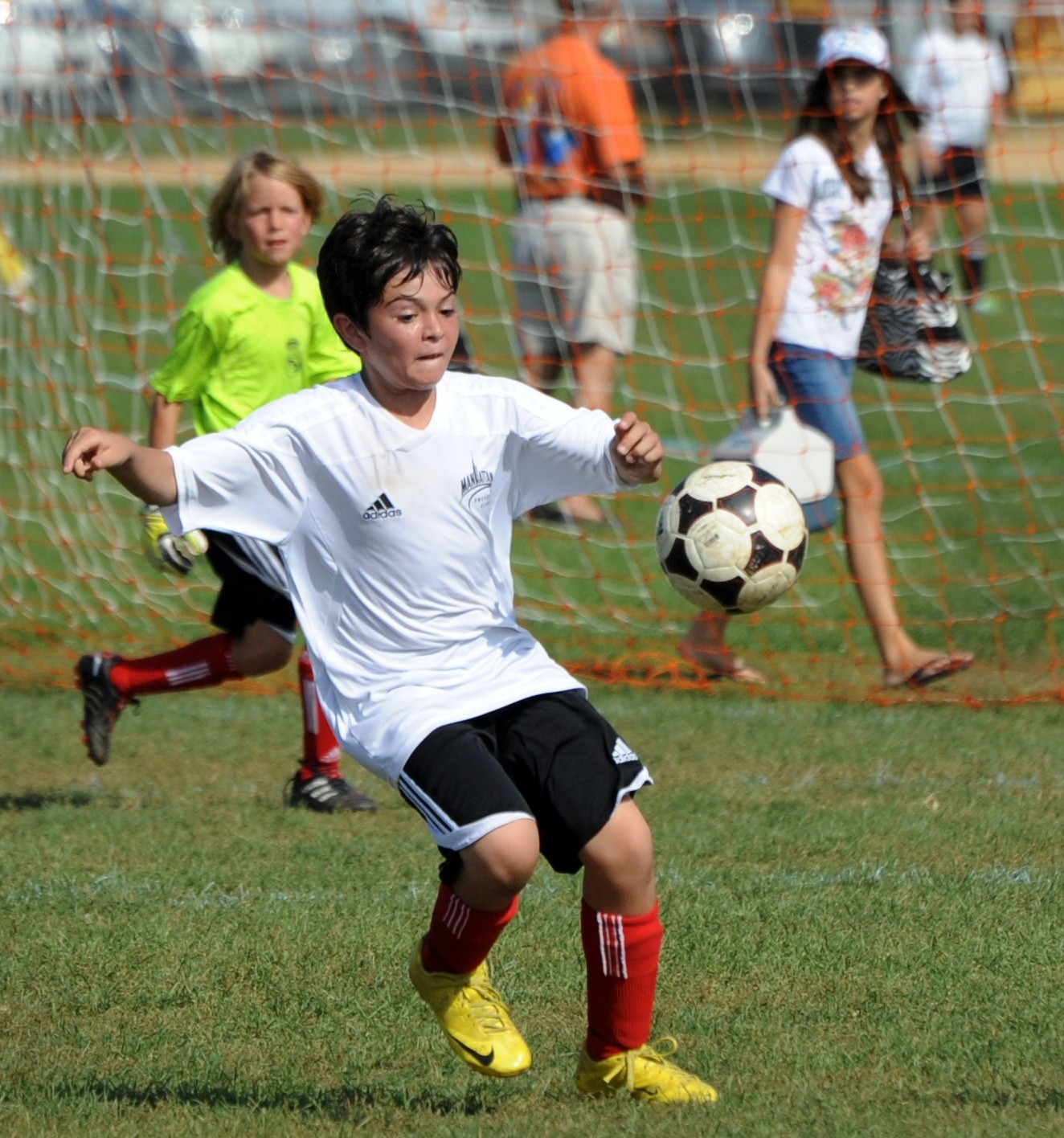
[655,462,809,615]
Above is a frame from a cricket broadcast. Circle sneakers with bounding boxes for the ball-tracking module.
[409,932,531,1077]
[75,651,142,768]
[284,768,378,815]
[573,1035,718,1106]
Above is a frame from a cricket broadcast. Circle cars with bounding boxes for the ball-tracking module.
[1,2,1064,125]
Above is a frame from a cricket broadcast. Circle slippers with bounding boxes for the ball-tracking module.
[678,641,765,682]
[889,656,967,690]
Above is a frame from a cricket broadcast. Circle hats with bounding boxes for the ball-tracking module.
[816,22,891,71]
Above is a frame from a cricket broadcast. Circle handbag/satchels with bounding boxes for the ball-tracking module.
[856,241,973,383]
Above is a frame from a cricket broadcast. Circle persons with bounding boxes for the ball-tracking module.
[905,0,1010,315]
[488,0,652,525]
[674,17,975,692]
[73,148,382,815]
[57,196,720,1107]
